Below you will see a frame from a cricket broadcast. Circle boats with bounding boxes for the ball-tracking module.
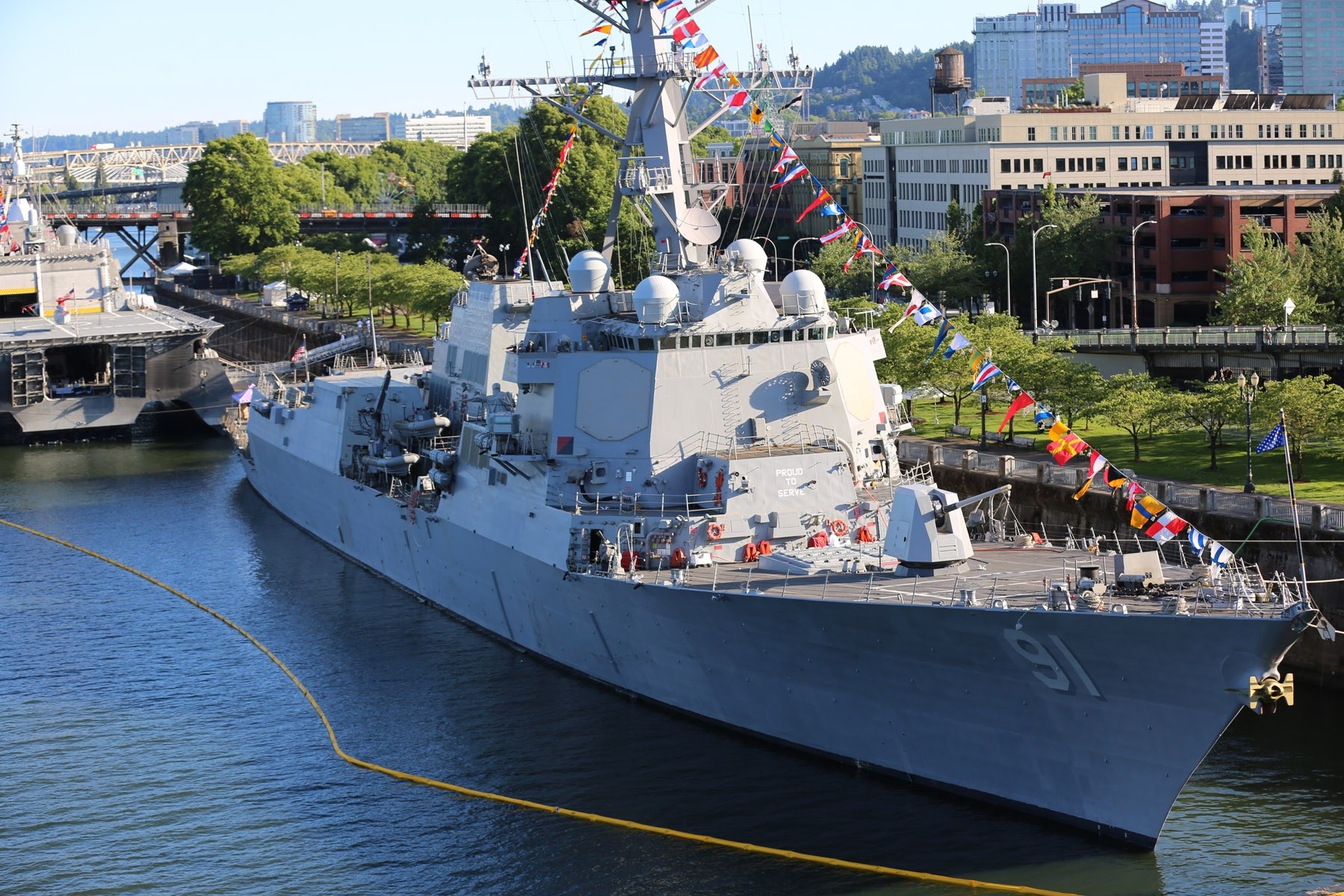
[394,412,451,439]
[0,123,241,446]
[361,448,421,477]
[237,1,1322,855]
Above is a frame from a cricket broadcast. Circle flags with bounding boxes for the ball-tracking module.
[1255,419,1285,455]
[55,288,75,301]
[648,0,1238,572]
[0,191,19,252]
[291,338,306,361]
[506,24,613,281]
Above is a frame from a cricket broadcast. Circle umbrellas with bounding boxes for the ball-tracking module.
[163,262,197,275]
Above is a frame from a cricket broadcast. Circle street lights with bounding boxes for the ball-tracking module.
[753,236,778,282]
[280,261,291,297]
[984,242,1011,330]
[1236,371,1260,494]
[1131,221,1157,344]
[334,249,341,298]
[1032,223,1059,334]
[498,243,511,278]
[979,347,992,450]
[792,237,819,271]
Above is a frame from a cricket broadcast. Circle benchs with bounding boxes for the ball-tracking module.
[977,431,1006,449]
[1003,435,1037,452]
[944,426,972,442]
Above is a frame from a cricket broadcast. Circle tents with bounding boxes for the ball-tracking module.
[283,293,309,311]
[263,281,308,306]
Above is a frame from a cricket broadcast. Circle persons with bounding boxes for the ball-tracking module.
[1265,324,1272,345]
[357,319,363,328]
[339,332,345,344]
[365,317,371,333]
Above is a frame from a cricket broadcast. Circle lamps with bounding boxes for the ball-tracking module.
[703,514,711,519]
[1016,610,1028,631]
[675,515,688,522]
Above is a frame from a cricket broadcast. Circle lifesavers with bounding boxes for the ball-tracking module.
[830,519,847,536]
[713,469,723,506]
[699,473,707,487]
[707,524,722,540]
